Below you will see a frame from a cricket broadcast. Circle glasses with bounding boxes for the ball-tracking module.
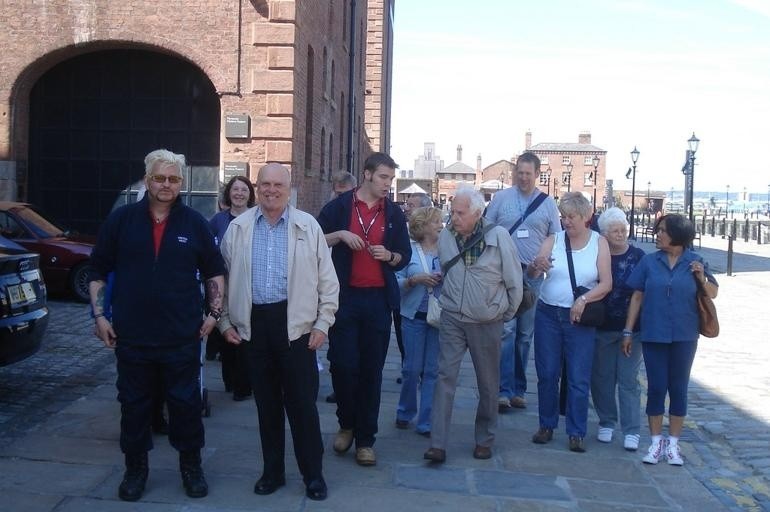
[147,174,184,184]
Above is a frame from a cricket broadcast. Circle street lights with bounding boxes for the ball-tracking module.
[686,132,701,250]
[629,146,640,241]
[500,154,602,216]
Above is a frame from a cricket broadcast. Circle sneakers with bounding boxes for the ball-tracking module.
[499,394,528,409]
[423,448,446,462]
[356,446,377,464]
[119,465,149,501]
[326,390,337,402]
[333,427,354,454]
[474,445,492,458]
[533,427,683,465]
[180,467,208,497]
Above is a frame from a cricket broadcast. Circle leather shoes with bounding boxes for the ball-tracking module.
[256,474,286,493]
[303,474,327,500]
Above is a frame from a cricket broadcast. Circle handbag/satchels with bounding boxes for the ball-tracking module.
[572,286,606,328]
[512,279,535,318]
[426,294,443,331]
[696,292,720,338]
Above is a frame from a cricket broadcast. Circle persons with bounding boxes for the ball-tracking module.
[83,152,561,468]
[214,161,341,501]
[526,190,719,468]
[88,147,227,501]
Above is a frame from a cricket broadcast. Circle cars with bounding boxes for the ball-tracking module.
[0,199,113,303]
[0,232,49,366]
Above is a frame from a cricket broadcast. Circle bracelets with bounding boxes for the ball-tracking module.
[206,311,221,324]
